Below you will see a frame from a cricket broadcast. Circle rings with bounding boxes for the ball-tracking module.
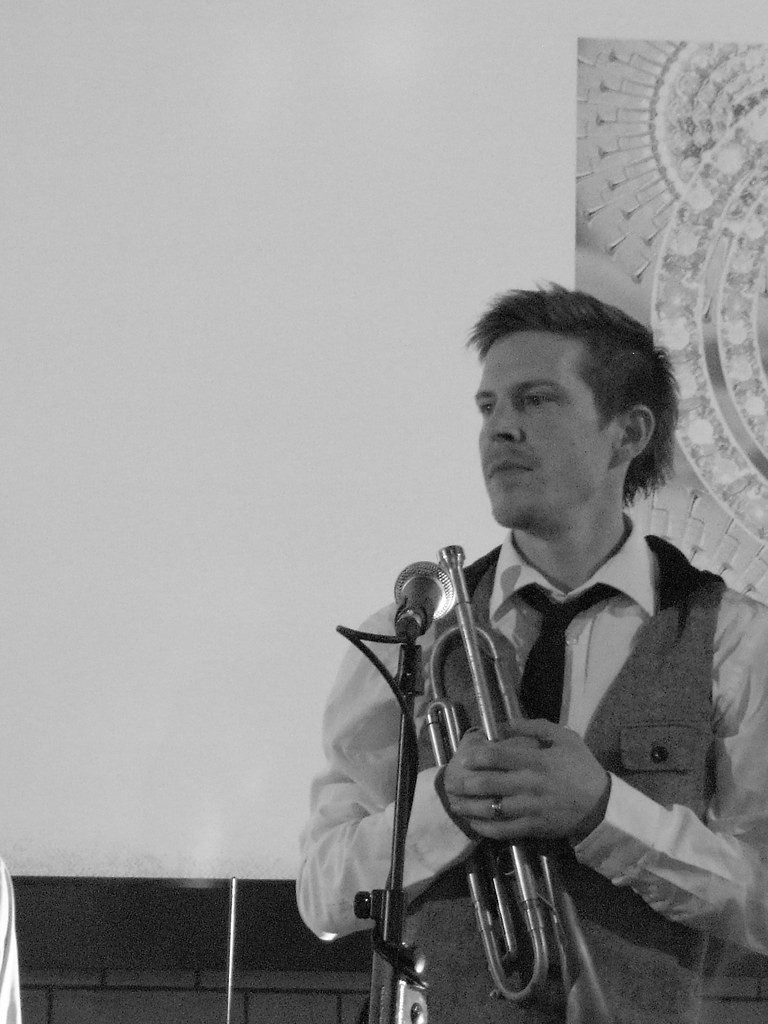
[492,796,503,823]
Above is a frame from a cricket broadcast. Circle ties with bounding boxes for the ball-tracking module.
[514,584,623,748]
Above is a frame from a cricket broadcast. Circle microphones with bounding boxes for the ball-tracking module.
[394,561,455,641]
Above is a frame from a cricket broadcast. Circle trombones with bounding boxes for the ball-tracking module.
[424,545,613,1024]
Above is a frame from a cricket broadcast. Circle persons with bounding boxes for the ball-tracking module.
[296,283,768,1024]
